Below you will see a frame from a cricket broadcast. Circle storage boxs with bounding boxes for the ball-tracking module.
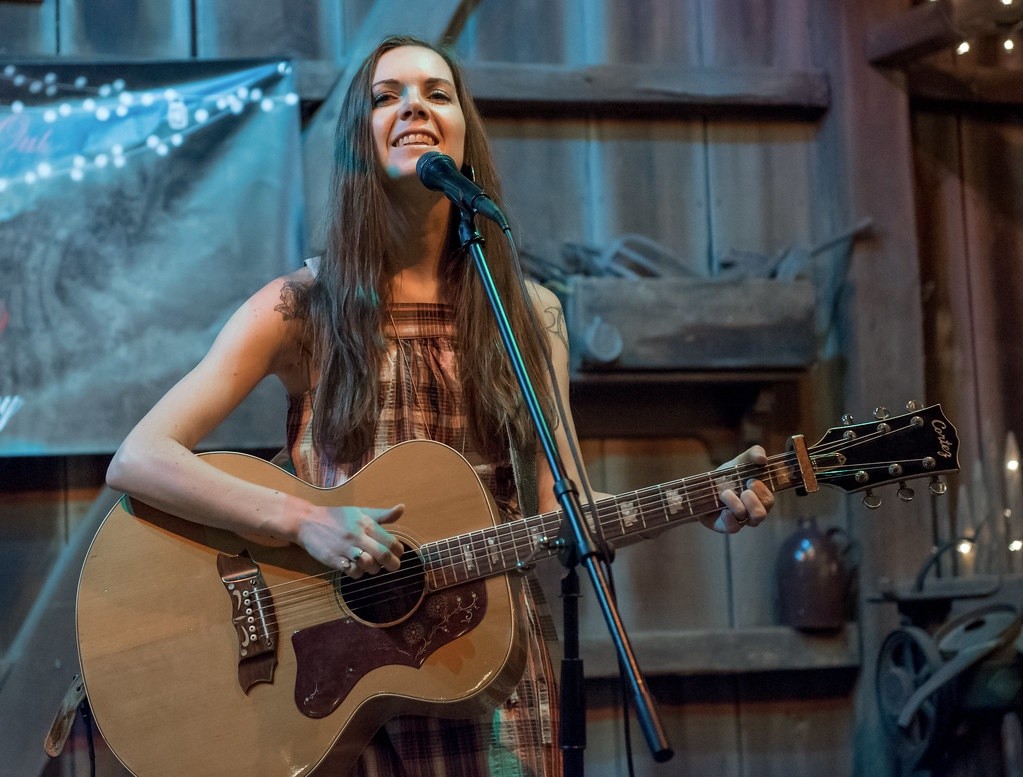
[563,275,819,371]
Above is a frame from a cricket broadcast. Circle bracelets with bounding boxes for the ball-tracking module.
[105,32,776,777]
[636,531,660,540]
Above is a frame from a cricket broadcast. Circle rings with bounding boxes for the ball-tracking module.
[739,517,748,523]
[341,560,351,573]
[352,550,364,561]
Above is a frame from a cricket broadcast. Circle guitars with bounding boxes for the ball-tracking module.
[73,401,965,777]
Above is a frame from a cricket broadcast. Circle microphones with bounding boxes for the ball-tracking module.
[416,151,513,235]
